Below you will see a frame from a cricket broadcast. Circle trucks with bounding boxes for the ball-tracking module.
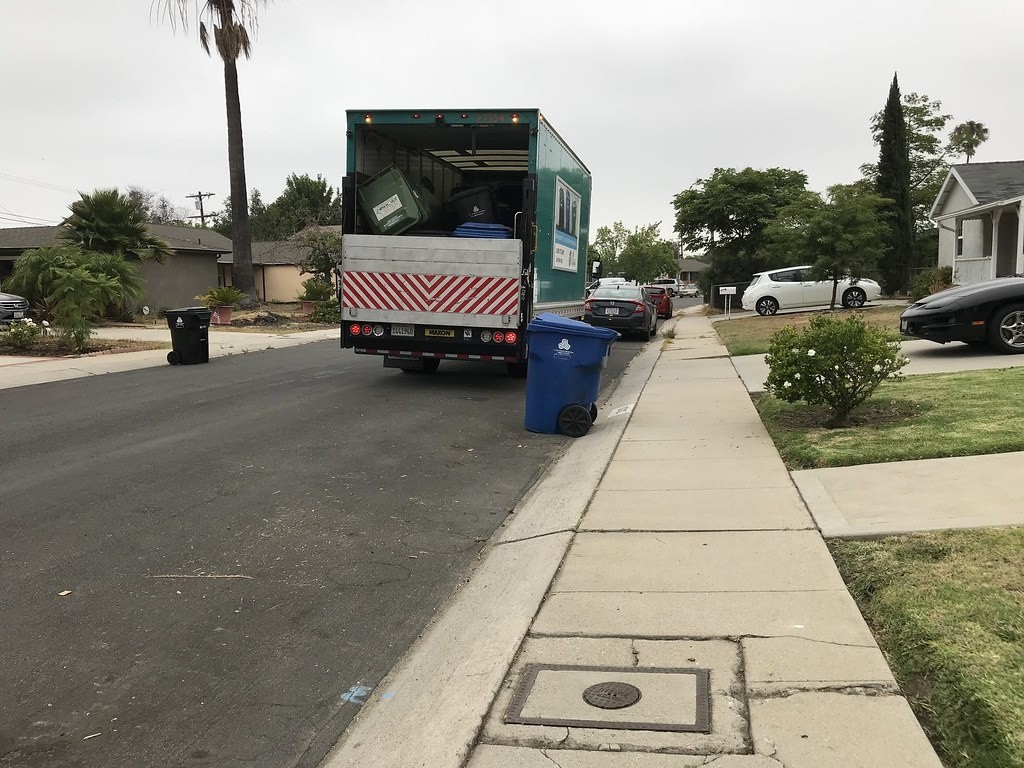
[340,107,603,380]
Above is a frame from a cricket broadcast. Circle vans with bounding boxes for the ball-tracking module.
[585,278,626,297]
[643,278,685,297]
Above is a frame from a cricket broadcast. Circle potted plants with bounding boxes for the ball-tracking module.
[298,279,332,313]
[193,286,245,324]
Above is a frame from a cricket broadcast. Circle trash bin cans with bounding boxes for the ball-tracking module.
[523,312,622,438]
[358,161,431,236]
[160,306,212,365]
[446,177,501,223]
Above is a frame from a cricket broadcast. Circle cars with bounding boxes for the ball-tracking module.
[584,284,662,342]
[899,271,1024,354]
[741,266,883,316]
[0,292,30,324]
[679,284,701,299]
[642,284,676,320]
